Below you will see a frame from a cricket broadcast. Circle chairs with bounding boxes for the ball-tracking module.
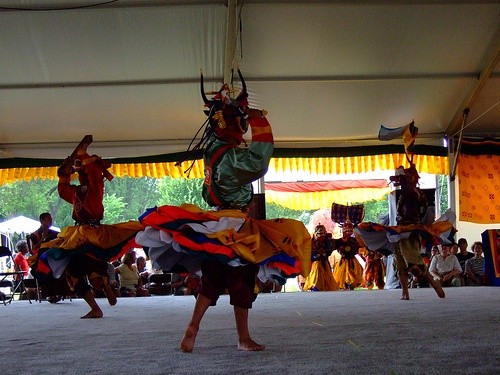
[0,280,15,301]
[148,273,173,296]
[19,279,38,300]
[35,277,73,303]
[0,245,32,306]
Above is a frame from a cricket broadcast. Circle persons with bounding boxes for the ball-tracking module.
[7,248,20,282]
[135,67,311,354]
[26,212,64,303]
[39,145,143,319]
[13,240,29,294]
[95,251,202,296]
[354,164,457,300]
[305,223,488,290]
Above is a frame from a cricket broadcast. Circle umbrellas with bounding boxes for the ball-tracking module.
[0,216,62,234]
